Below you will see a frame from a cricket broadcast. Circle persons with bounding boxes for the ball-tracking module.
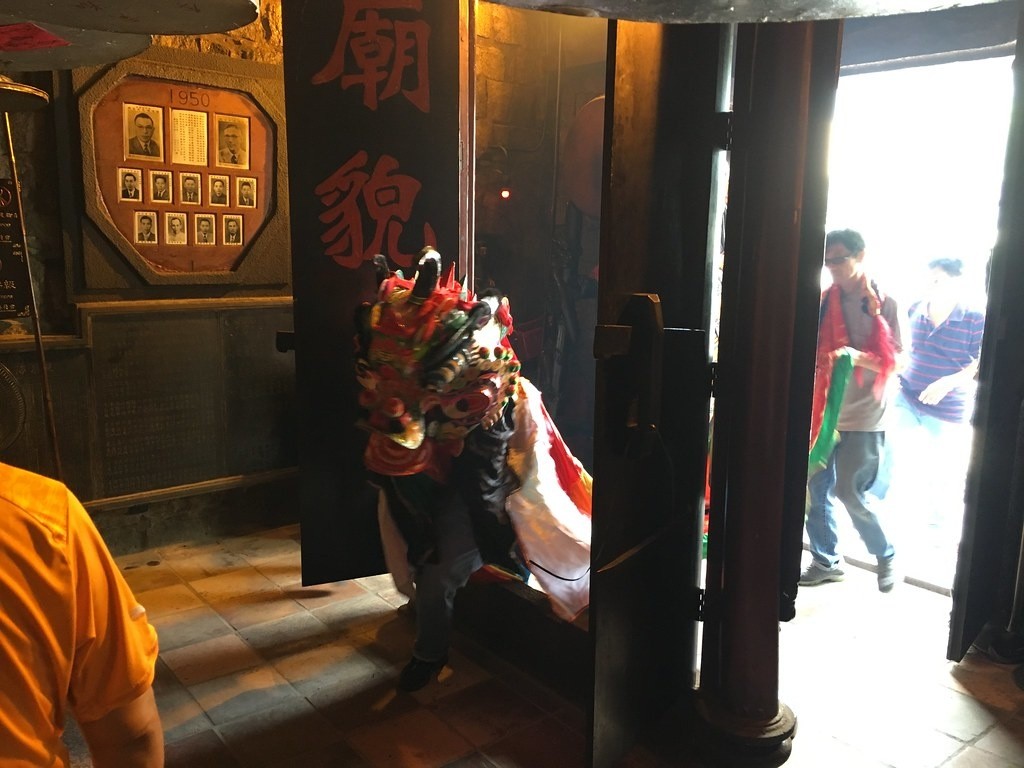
[0,461,165,768]
[211,179,227,204]
[153,176,170,200]
[197,218,213,242]
[375,424,530,693]
[121,173,139,199]
[168,217,185,243]
[138,215,155,242]
[864,257,984,500]
[239,182,253,206]
[182,177,198,202]
[796,228,904,594]
[225,219,240,244]
[219,124,247,165]
[129,112,160,157]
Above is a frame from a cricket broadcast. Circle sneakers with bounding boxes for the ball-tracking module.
[797,561,848,586]
[877,552,895,595]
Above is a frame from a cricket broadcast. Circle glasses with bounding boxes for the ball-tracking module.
[225,134,240,139]
[135,124,153,130]
[824,252,857,267]
